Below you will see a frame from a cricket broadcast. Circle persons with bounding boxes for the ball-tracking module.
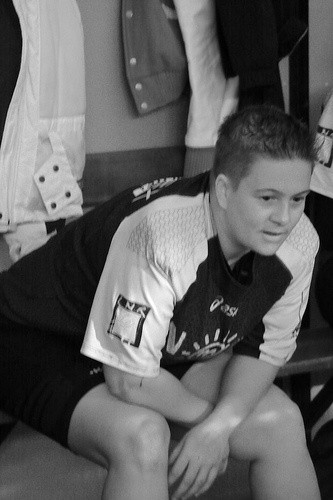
[0,107,322,500]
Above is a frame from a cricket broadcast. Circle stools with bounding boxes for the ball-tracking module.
[0,412,256,500]
